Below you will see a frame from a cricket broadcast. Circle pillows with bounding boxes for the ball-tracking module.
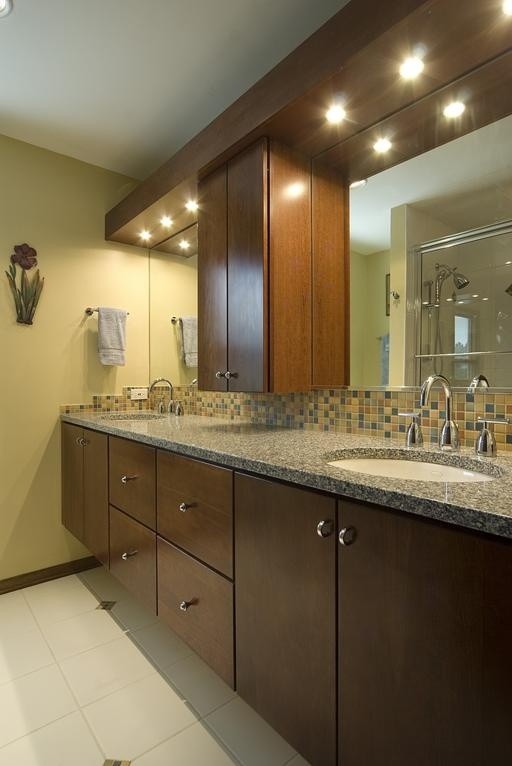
[178,317,198,368]
[97,305,127,369]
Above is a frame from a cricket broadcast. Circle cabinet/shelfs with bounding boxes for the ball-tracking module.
[61,421,109,576]
[311,159,351,391]
[108,435,158,617]
[157,448,236,692]
[234,470,511,766]
[198,133,312,392]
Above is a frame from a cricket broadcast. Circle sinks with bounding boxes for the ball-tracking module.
[320,448,504,483]
[99,414,165,422]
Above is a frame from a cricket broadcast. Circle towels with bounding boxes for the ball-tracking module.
[381,333,390,388]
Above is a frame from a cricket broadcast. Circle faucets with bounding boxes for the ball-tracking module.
[188,379,197,386]
[418,374,461,450]
[150,378,174,413]
[467,376,489,391]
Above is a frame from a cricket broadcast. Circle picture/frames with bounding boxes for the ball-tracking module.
[385,273,390,316]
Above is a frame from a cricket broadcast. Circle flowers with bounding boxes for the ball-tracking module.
[5,242,48,328]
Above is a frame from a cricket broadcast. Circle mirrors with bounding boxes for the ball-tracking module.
[313,44,511,397]
[148,222,199,387]
